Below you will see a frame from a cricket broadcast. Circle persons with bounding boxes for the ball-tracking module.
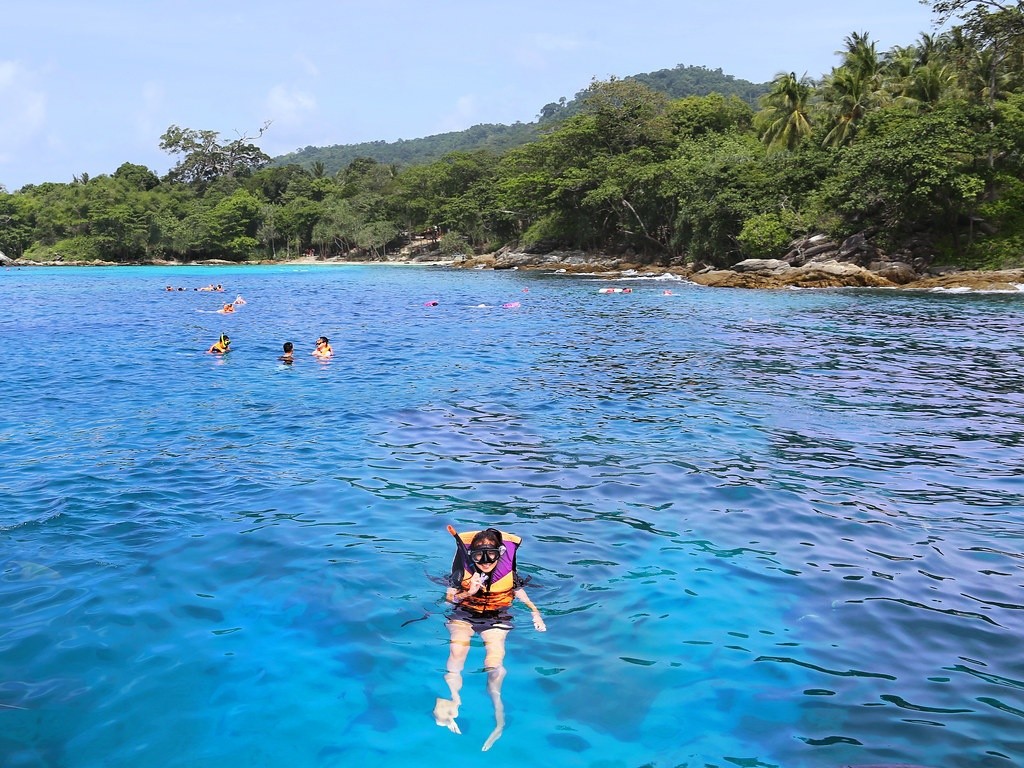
[165,282,246,316]
[312,336,333,356]
[447,527,523,594]
[282,342,294,357]
[208,332,231,354]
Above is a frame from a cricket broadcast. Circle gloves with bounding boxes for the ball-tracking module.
[468,572,489,595]
[532,611,546,632]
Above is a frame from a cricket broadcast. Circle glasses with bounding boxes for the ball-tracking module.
[470,544,501,564]
[316,341,325,345]
